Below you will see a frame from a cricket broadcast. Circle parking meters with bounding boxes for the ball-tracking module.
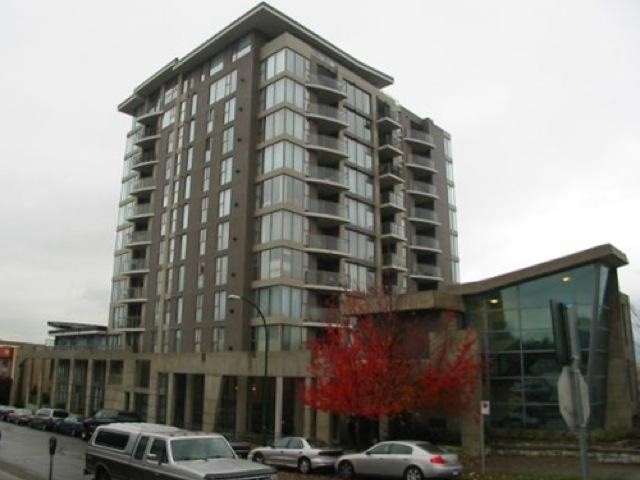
[48,437,57,480]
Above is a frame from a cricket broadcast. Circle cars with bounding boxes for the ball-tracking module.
[0,405,82,437]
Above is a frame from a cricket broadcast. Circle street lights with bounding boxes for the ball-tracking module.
[228,294,269,446]
